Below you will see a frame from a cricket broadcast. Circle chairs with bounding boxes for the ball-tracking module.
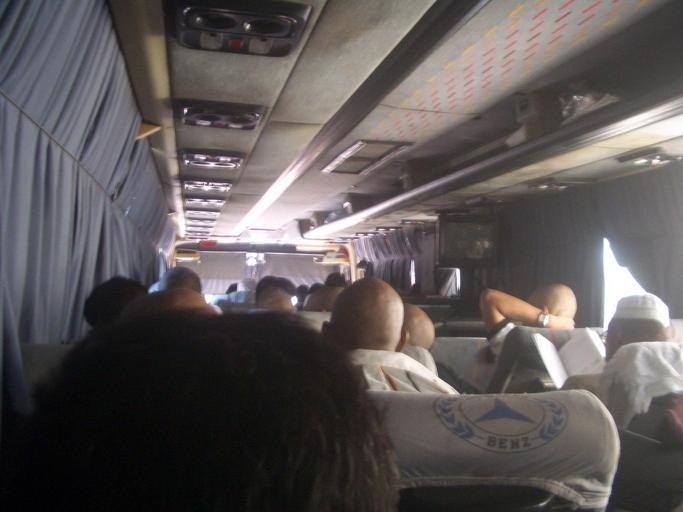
[201,288,680,511]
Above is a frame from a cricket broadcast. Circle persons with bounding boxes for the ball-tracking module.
[1,267,682,510]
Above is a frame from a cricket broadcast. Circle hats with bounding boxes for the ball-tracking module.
[614,294,671,327]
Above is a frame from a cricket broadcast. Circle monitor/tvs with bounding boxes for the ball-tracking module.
[437,210,503,266]
